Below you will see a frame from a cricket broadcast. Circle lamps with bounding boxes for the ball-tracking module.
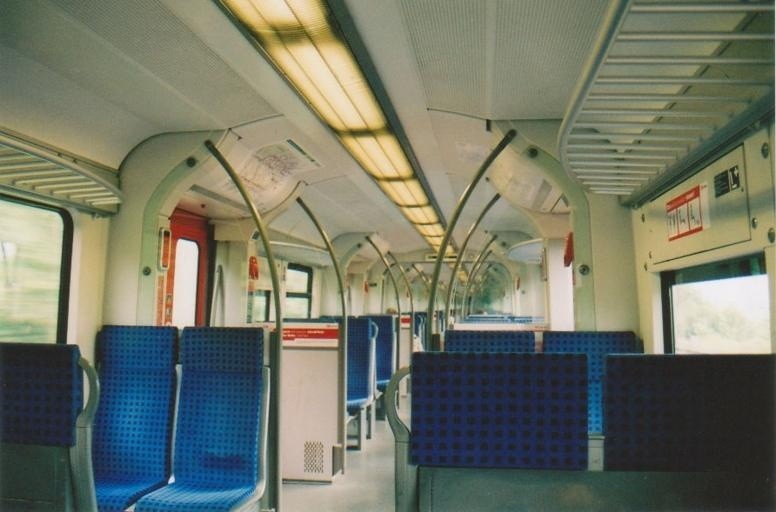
[214,0,458,261]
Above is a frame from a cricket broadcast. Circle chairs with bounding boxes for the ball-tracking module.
[3,324,266,510]
[281,314,396,453]
[384,308,774,510]
